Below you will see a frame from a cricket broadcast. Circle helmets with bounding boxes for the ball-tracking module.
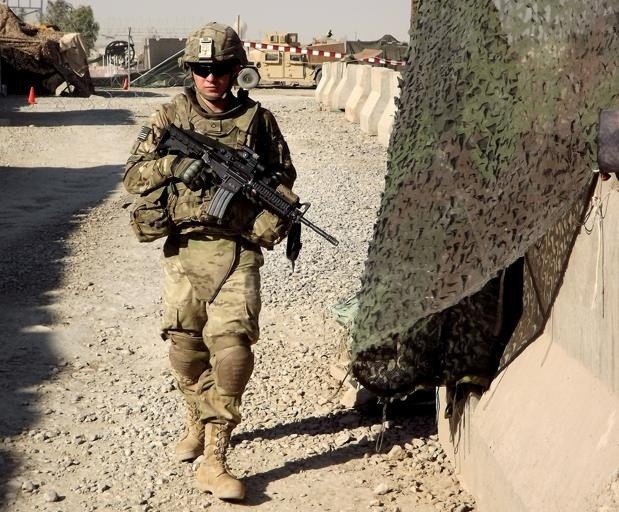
[178,21,249,68]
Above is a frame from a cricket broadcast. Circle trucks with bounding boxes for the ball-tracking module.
[236,32,322,90]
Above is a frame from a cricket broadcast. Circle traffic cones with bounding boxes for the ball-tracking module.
[122,78,128,90]
[26,86,37,104]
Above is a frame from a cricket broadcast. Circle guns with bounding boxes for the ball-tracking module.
[154,123,339,247]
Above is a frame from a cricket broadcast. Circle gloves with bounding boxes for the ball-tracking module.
[243,184,264,207]
[172,157,214,192]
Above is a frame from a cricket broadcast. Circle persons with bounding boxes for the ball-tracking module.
[123,20,300,501]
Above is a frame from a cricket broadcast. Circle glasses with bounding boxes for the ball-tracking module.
[191,65,234,79]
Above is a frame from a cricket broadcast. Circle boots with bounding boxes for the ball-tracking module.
[176,376,246,500]
